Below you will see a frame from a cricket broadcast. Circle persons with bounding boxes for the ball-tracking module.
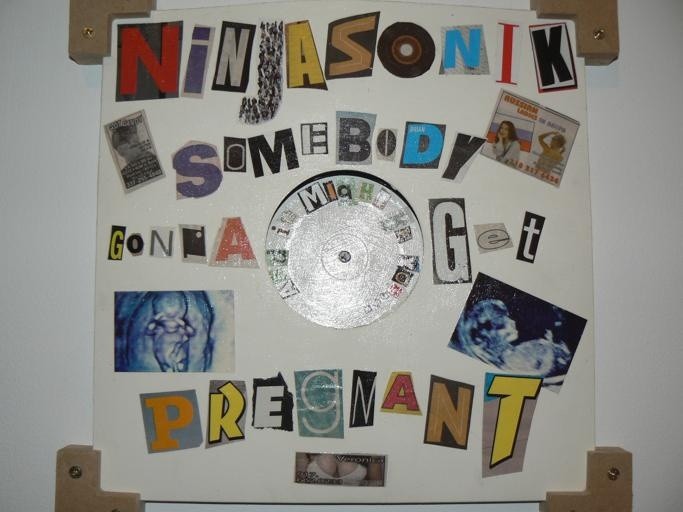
[535,131,566,174]
[458,298,571,378]
[111,125,142,161]
[495,121,521,164]
